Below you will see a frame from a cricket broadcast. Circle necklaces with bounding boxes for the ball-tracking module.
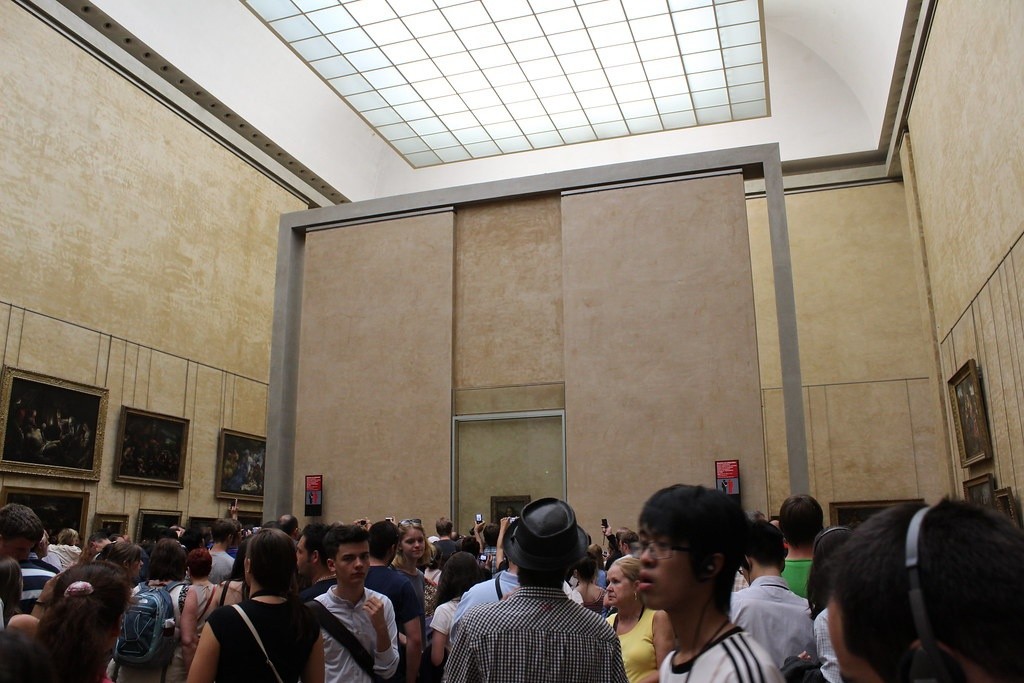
[686,619,729,683]
[314,574,337,584]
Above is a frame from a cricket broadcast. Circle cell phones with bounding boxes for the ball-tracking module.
[476,514,482,524]
[509,517,520,524]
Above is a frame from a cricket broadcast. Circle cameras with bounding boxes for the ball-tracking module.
[386,518,394,522]
[361,520,367,525]
[480,556,488,561]
[601,518,608,528]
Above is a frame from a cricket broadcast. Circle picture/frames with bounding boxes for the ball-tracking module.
[962,473,1019,530]
[830,498,925,532]
[947,358,993,468]
[0,364,266,545]
[490,495,531,525]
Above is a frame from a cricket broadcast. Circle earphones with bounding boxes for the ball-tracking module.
[707,565,715,573]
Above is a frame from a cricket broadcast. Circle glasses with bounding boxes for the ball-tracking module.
[637,540,694,561]
[399,519,422,526]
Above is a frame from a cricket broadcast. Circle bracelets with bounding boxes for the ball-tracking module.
[35,600,46,606]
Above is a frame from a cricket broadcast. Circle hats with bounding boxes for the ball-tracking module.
[503,497,589,573]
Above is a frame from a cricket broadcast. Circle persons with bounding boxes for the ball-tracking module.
[636,484,785,683]
[0,498,1024,683]
[722,480,733,493]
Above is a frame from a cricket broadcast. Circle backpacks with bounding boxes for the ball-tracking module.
[112,581,187,671]
